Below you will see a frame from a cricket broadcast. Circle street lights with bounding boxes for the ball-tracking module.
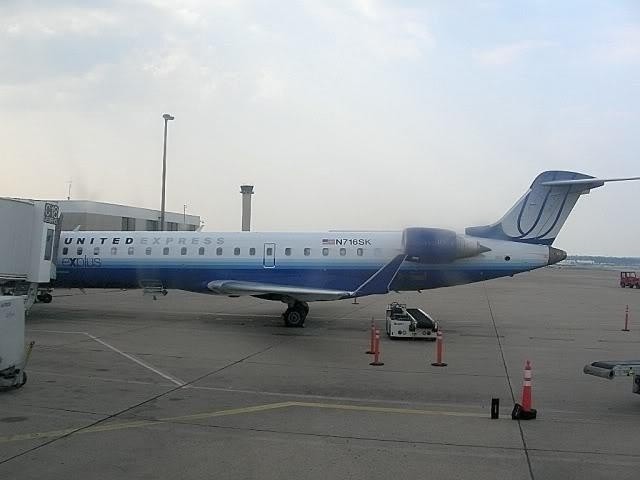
[160,112,177,231]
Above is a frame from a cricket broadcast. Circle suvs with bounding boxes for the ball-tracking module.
[620,270,640,290]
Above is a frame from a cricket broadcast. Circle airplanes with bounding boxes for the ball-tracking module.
[49,167,640,331]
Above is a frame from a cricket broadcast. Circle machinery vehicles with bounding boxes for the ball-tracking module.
[383,299,439,343]
